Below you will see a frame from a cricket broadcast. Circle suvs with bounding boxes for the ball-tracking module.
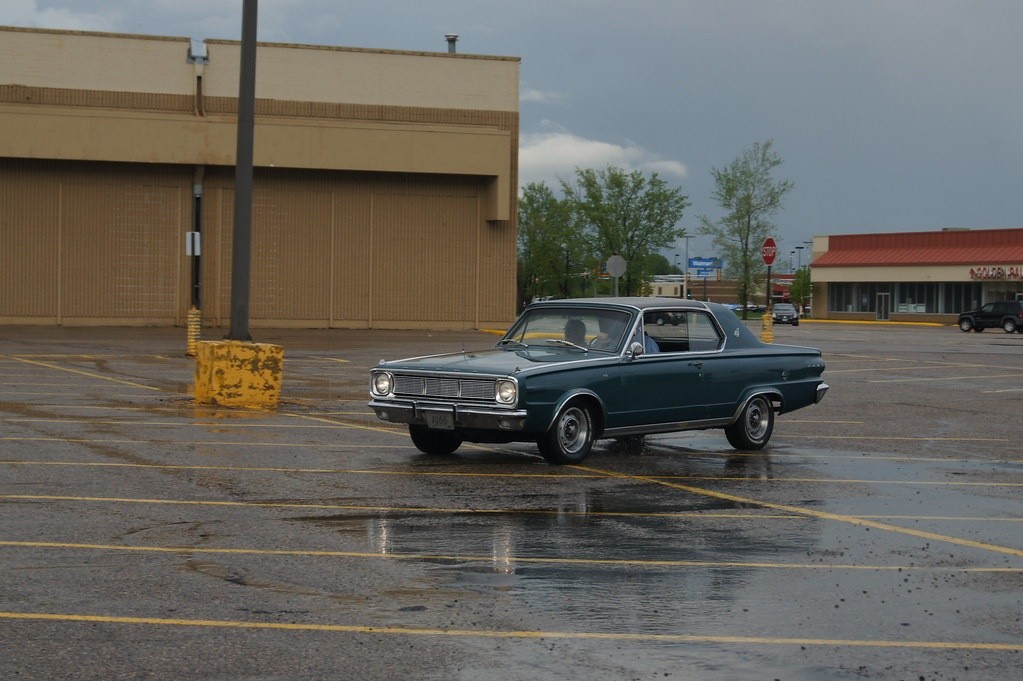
[958,301,1023,334]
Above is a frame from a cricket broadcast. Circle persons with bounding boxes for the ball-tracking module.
[597,312,660,353]
[562,319,589,348]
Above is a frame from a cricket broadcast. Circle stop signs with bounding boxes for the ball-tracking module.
[761,238,777,267]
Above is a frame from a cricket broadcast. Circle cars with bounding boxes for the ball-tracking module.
[795,302,811,317]
[773,303,798,326]
[722,303,767,314]
[367,296,830,466]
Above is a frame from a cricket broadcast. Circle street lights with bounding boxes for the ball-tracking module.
[680,235,696,299]
